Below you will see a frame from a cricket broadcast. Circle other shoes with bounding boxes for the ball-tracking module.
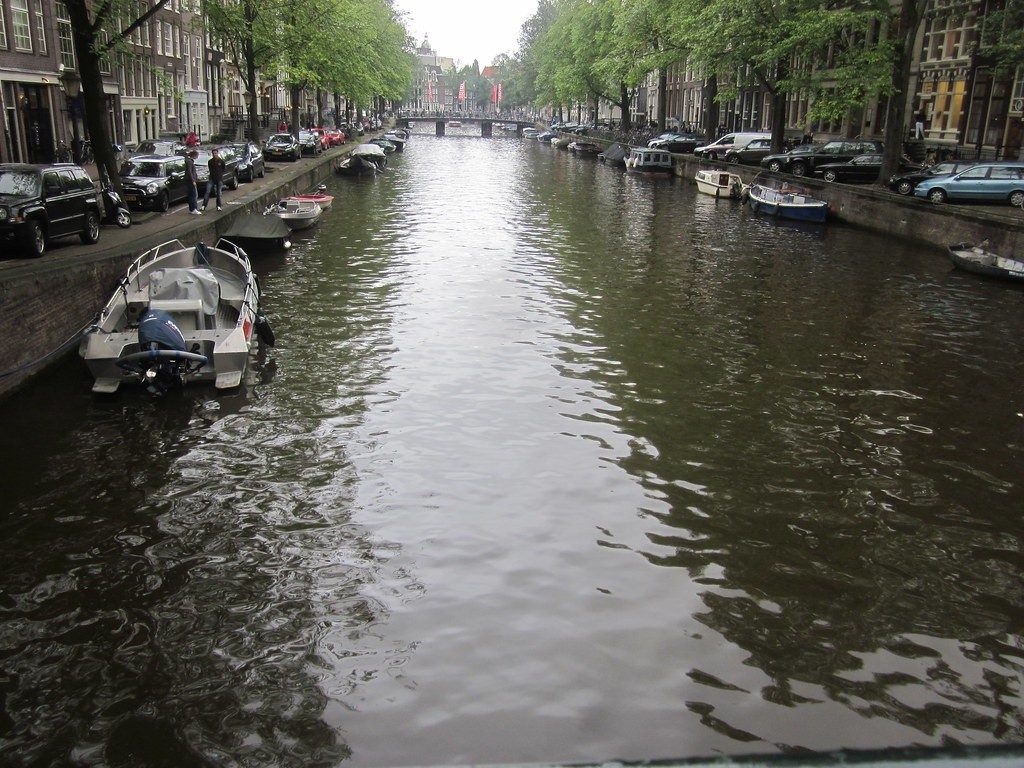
[191,209,202,215]
[217,206,222,211]
[198,206,205,210]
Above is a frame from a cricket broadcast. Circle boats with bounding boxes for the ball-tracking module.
[695,169,751,200]
[214,184,333,261]
[748,183,827,223]
[945,241,1024,278]
[78,237,260,420]
[623,148,674,178]
[339,128,409,175]
[496,124,627,168]
[449,120,462,128]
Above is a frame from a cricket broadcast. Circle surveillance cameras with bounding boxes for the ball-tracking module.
[59,64,64,72]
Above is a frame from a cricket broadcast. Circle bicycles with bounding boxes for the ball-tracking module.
[55,138,94,165]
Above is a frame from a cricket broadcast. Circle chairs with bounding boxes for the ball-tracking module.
[288,201,299,212]
[147,298,206,333]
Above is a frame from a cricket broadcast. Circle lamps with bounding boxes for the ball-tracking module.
[144,105,150,115]
[21,96,30,108]
[264,92,270,100]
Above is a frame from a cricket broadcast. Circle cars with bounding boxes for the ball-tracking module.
[119,139,190,212]
[760,139,920,185]
[339,119,381,138]
[263,128,345,163]
[551,122,586,136]
[188,140,266,198]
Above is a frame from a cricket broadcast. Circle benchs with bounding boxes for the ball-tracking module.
[785,192,811,201]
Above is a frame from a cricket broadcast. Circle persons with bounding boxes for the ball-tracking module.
[279,122,287,133]
[184,151,201,215]
[915,109,925,140]
[200,150,225,211]
[800,132,812,146]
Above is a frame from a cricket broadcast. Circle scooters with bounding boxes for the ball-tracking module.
[99,163,131,229]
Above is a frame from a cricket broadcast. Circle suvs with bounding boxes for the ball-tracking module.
[888,160,1024,208]
[0,163,100,259]
[646,131,790,165]
